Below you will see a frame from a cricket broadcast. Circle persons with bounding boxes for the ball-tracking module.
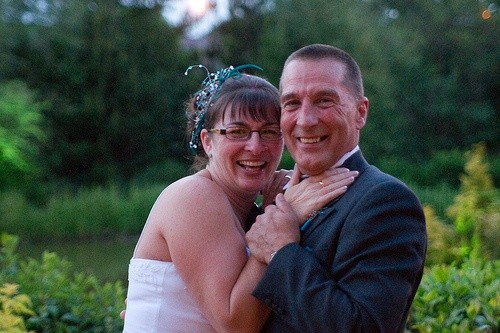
[119,43,428,333]
[121,62,360,333]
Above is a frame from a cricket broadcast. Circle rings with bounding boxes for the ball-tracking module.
[317,181,325,190]
[284,174,290,179]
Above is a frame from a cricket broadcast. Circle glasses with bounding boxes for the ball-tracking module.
[208,126,284,141]
